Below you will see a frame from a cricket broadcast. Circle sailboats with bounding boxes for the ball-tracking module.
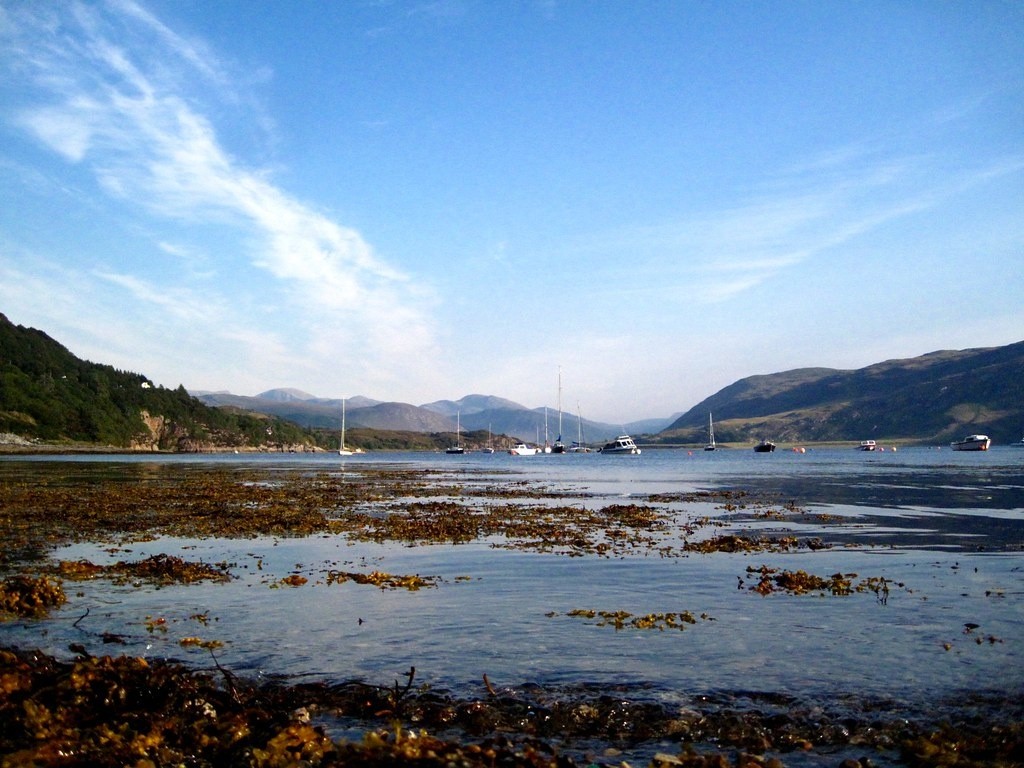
[705,412,715,451]
[483,423,495,454]
[534,365,591,454]
[446,410,465,454]
[338,396,353,456]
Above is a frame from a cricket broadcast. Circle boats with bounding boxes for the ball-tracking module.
[860,440,876,451]
[507,442,537,456]
[754,442,775,453]
[950,435,992,451]
[601,436,641,454]
